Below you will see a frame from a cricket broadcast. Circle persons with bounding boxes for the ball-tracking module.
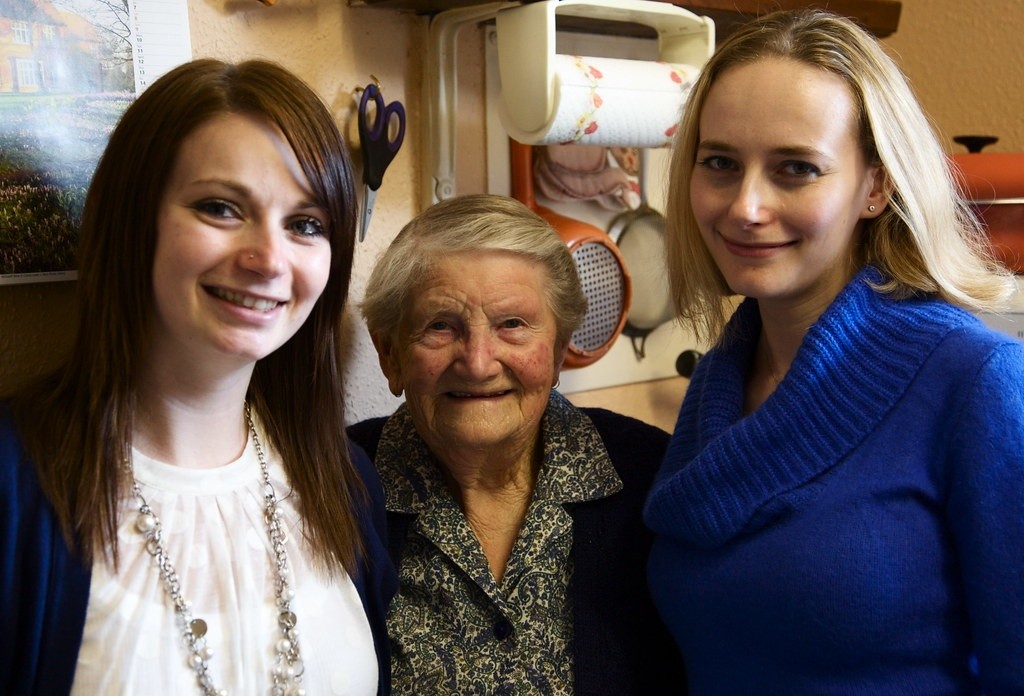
[631,7,1024,696]
[344,193,673,696]
[0,56,392,695]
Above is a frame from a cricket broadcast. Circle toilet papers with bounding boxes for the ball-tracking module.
[495,54,698,149]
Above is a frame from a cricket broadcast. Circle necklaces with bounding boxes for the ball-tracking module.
[118,402,308,696]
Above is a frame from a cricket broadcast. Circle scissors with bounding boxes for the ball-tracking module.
[358,83,407,242]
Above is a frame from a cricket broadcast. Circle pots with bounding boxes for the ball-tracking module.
[510,136,634,372]
[941,133,1024,275]
